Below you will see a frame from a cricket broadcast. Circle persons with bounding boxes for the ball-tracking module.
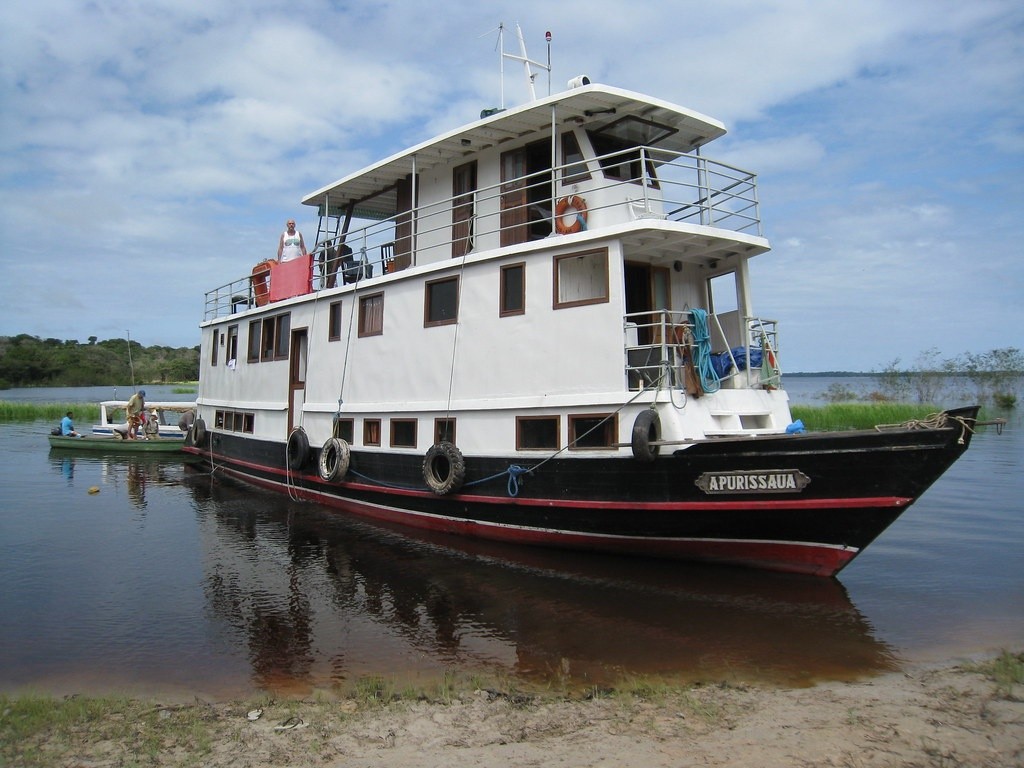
[125,390,145,439]
[178,409,197,438]
[142,409,160,440]
[60,412,85,438]
[278,219,306,263]
[319,240,340,289]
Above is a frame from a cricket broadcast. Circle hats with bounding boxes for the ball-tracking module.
[149,409,158,419]
[323,240,332,246]
[139,390,145,397]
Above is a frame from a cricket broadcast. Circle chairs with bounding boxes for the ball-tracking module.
[340,246,366,285]
[381,242,396,276]
[231,277,254,314]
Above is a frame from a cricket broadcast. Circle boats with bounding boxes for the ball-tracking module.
[90,399,197,437]
[48,432,184,452]
[182,23,1004,581]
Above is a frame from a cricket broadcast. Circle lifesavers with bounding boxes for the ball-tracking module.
[191,420,206,447]
[288,430,311,470]
[554,194,588,235]
[318,437,350,482]
[424,441,466,496]
[674,319,692,357]
[632,409,661,460]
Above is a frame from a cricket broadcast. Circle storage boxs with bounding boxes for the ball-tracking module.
[346,261,373,278]
